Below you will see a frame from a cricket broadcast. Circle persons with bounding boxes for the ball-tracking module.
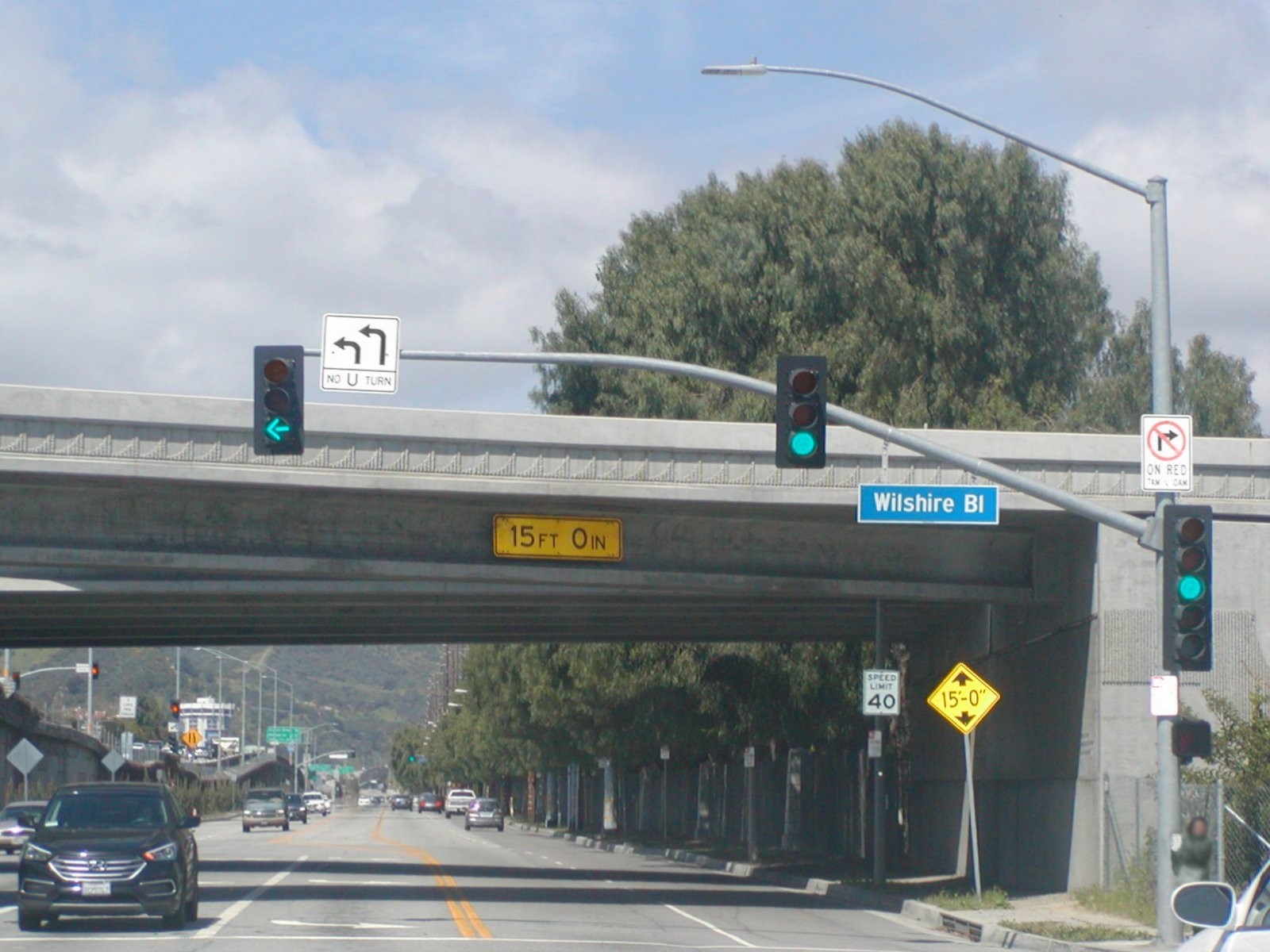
[138,802,163,828]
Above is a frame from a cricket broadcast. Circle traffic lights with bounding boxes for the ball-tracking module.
[171,700,180,718]
[1174,717,1212,762]
[409,746,415,762]
[11,671,21,690]
[773,354,829,472]
[1163,504,1213,672]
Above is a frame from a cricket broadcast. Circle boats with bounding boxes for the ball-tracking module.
[92,663,99,680]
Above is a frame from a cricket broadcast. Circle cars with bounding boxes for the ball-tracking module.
[283,791,333,824]
[357,793,445,814]
[445,788,479,818]
[242,787,291,832]
[463,798,505,832]
[128,728,242,762]
[0,800,54,855]
[1167,861,1270,951]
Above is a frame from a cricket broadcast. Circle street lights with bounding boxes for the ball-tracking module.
[243,660,278,745]
[700,62,1181,938]
[192,645,222,773]
[216,655,262,762]
[261,675,293,767]
[293,722,339,797]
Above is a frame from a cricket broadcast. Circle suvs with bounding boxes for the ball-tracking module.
[18,780,203,932]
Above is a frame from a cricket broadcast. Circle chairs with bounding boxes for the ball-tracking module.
[117,799,163,826]
[63,797,104,828]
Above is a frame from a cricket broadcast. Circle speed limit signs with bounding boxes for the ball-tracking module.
[861,669,902,716]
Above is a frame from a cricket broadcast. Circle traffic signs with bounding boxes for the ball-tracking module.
[321,312,400,397]
[252,346,309,458]
